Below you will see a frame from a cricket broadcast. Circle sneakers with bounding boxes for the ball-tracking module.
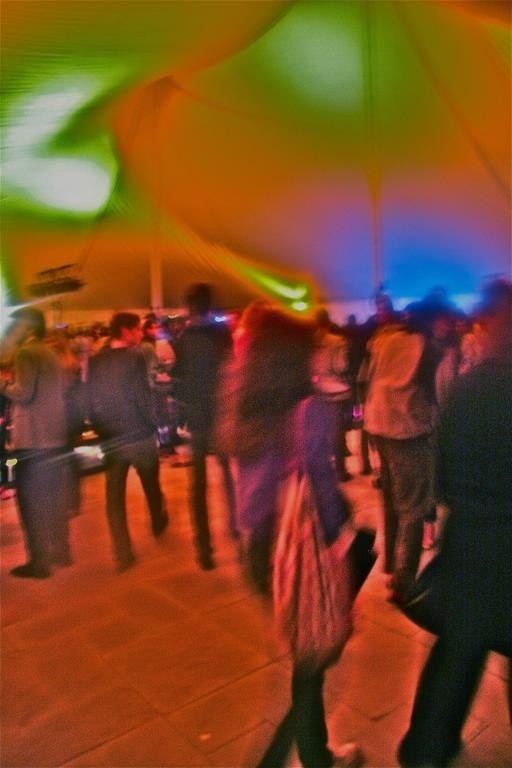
[330,741,358,768]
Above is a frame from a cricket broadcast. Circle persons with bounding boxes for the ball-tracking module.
[2,274,512,767]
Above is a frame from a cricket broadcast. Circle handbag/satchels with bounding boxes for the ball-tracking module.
[271,472,352,669]
